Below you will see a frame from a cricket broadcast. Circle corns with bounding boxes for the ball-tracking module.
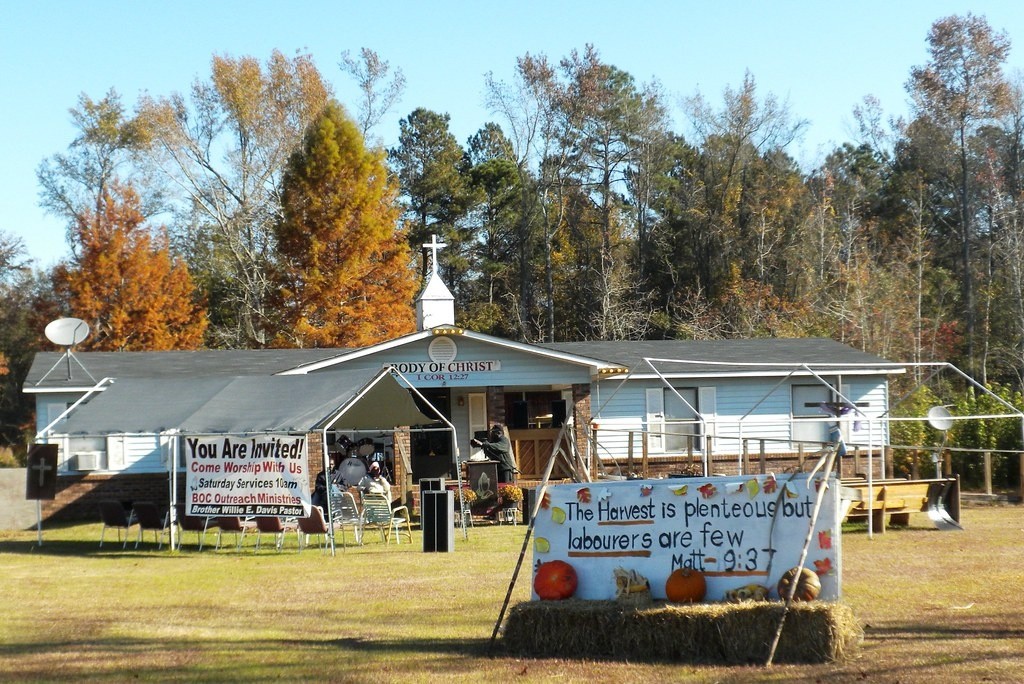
[617,576,648,592]
[742,585,766,601]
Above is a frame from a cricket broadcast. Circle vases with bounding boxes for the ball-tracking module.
[459,502,470,510]
[504,500,518,508]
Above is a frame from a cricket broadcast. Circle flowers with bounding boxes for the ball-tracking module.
[500,484,524,501]
[455,489,477,502]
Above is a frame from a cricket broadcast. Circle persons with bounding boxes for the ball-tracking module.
[356,461,392,505]
[469,423,515,483]
[314,458,349,518]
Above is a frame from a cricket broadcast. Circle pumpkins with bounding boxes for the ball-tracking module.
[534,560,577,600]
[666,568,705,603]
[778,567,822,602]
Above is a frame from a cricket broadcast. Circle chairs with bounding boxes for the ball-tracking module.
[216,516,257,553]
[100,501,140,550]
[177,514,218,550]
[329,492,363,546]
[135,506,171,549]
[255,517,300,552]
[297,506,330,552]
[362,494,413,544]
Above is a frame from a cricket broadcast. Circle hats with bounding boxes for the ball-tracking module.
[369,461,379,472]
[490,425,503,432]
